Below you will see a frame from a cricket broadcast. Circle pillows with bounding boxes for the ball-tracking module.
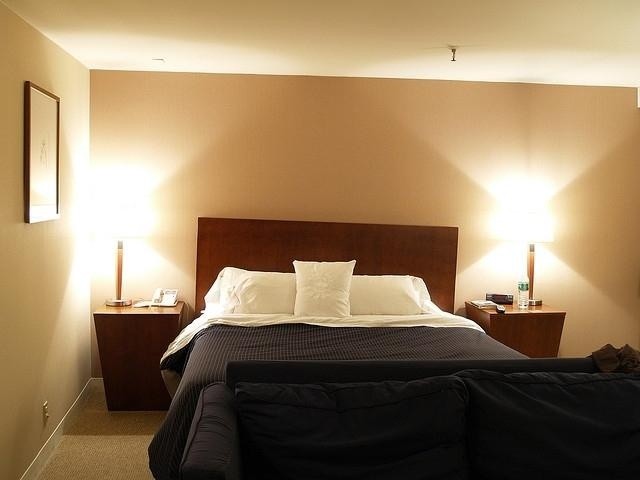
[204,267,432,315]
[454,369,639,480]
[234,374,469,480]
[292,257,357,318]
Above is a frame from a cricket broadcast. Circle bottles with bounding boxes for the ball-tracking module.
[518,276,530,309]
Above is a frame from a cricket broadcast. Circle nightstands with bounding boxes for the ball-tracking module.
[93,297,185,411]
[464,299,566,358]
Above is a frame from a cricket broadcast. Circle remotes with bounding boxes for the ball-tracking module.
[496,305,505,313]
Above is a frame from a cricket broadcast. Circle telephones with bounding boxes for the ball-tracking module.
[152,289,178,307]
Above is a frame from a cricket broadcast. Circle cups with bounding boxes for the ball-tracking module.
[132,298,146,308]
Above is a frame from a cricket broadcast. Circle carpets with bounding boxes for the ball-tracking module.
[37,389,168,480]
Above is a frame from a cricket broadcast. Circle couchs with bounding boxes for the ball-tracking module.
[175,359,639,480]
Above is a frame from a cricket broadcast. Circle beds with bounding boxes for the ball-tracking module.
[148,216,534,480]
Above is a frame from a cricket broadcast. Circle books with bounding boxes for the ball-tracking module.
[470,301,497,310]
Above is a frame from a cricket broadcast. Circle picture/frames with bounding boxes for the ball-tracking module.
[23,80,61,224]
